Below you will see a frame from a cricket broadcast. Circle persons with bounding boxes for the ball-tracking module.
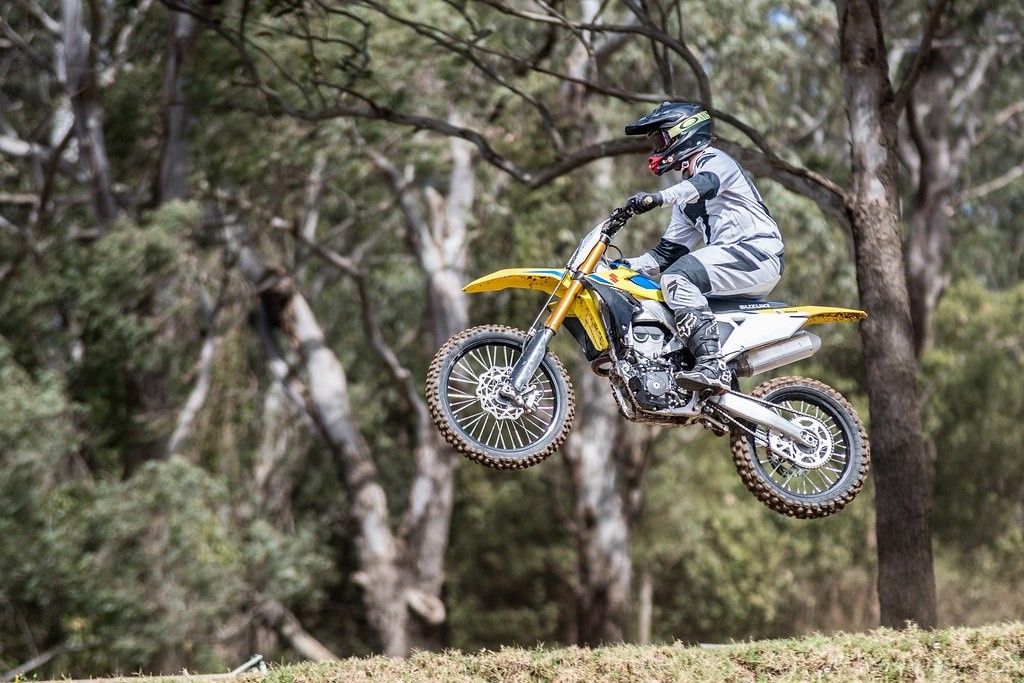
[612,104,785,393]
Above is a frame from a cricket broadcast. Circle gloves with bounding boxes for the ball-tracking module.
[626,192,663,215]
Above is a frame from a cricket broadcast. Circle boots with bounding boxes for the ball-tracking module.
[674,305,733,395]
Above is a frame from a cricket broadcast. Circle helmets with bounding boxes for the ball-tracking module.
[624,101,713,176]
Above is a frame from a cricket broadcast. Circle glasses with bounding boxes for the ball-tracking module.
[646,131,673,154]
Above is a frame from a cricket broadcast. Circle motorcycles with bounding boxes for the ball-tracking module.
[425,194,872,521]
